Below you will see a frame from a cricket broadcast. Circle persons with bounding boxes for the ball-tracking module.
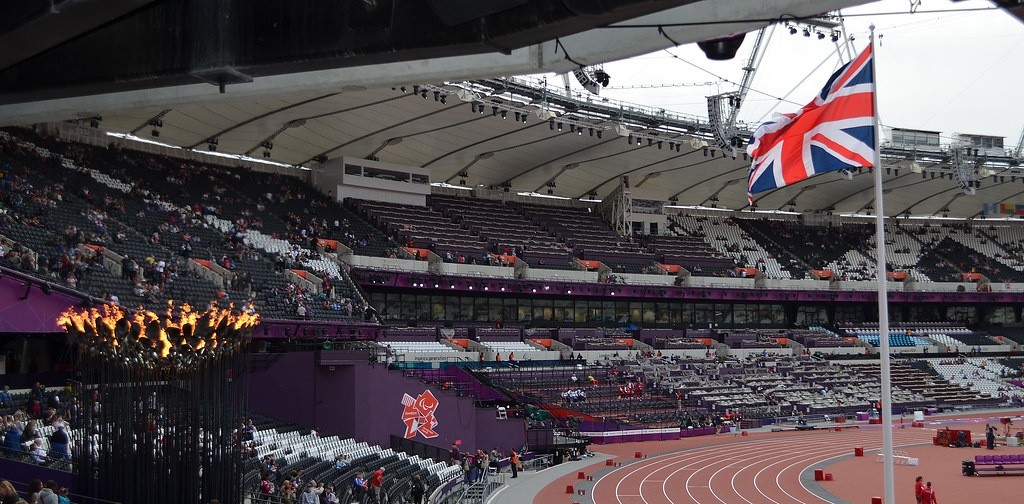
[0,123,1023,504]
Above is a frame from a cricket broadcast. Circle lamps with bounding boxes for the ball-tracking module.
[549,121,555,129]
[569,124,576,133]
[577,127,583,135]
[847,166,899,176]
[658,97,757,163]
[557,122,563,131]
[596,130,603,139]
[588,128,594,136]
[470,102,527,123]
[636,137,642,146]
[588,191,987,219]
[646,139,653,148]
[305,151,558,193]
[628,136,633,145]
[993,175,1024,185]
[392,84,447,105]
[779,21,842,43]
[88,114,274,155]
[353,262,986,302]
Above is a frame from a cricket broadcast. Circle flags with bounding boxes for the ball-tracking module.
[747,42,876,204]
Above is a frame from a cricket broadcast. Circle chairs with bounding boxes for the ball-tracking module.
[0,317,1024,504]
